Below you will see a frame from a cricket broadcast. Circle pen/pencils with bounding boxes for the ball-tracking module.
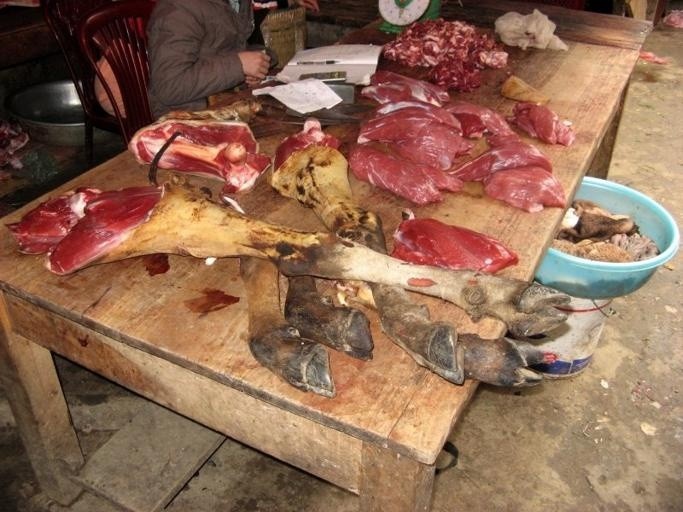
[297,60,338,64]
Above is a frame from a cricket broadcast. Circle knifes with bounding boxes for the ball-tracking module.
[256,96,376,125]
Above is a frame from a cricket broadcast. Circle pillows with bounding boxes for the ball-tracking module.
[94,39,148,118]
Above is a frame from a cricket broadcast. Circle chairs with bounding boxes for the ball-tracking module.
[77,0,154,153]
[41,0,119,167]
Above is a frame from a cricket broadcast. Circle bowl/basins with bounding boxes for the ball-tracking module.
[534,174,680,301]
[2,76,116,148]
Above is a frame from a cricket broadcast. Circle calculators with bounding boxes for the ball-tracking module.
[299,71,347,83]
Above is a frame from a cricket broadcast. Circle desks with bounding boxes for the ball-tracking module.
[0,0,654,512]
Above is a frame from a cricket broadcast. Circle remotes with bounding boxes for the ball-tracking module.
[298,70,347,82]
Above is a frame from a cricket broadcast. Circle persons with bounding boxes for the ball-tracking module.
[148,0,279,123]
[252,0,320,15]
